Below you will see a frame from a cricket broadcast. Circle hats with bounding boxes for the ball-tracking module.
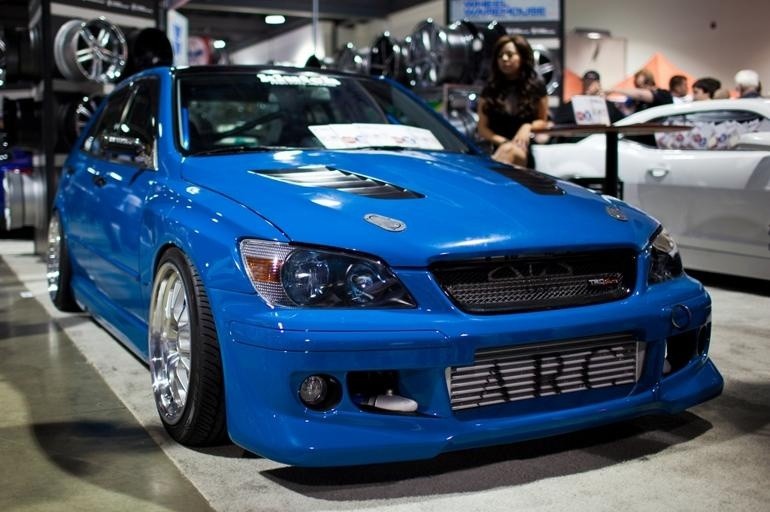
[735,69,759,88]
[693,78,720,96]
[580,72,600,81]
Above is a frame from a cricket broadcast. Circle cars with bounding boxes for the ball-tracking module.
[43,64,726,472]
[530,96,770,281]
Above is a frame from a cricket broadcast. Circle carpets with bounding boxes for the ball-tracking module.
[0,239,768,512]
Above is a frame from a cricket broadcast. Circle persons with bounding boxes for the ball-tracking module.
[730,69,763,98]
[554,70,627,143]
[691,77,720,101]
[669,74,690,103]
[601,66,673,113]
[477,32,549,169]
